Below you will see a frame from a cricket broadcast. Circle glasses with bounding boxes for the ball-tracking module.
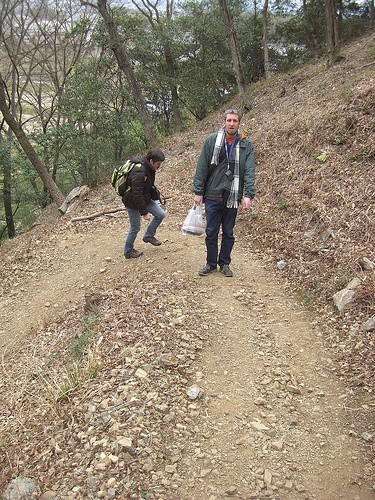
[225,108,239,114]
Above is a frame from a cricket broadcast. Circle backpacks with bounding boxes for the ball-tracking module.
[111,159,148,196]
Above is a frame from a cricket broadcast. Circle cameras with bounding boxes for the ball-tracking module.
[225,170,234,181]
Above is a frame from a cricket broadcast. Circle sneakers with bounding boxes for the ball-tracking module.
[220,264,233,277]
[198,264,217,276]
[125,249,143,259]
[142,236,162,246]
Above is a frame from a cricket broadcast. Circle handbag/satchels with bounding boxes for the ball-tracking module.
[150,185,166,205]
